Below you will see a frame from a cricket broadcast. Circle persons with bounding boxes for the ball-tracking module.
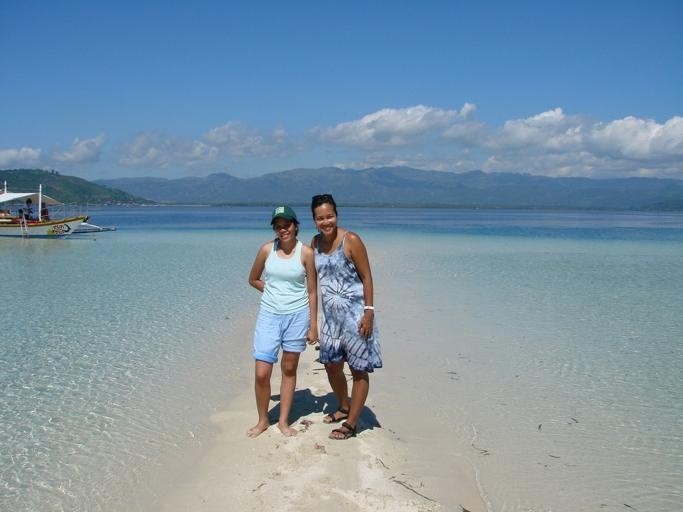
[21,198,33,220]
[307,193,384,441]
[244,205,319,440]
[39,202,48,220]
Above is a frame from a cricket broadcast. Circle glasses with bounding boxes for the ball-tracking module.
[272,223,291,230]
[312,194,332,200]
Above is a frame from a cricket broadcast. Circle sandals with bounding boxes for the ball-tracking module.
[324,405,350,423]
[328,420,357,439]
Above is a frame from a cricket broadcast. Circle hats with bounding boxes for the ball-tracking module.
[271,205,300,225]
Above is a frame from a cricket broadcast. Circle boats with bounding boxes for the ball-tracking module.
[0,180,89,240]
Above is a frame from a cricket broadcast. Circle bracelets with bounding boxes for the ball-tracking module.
[362,305,374,312]
